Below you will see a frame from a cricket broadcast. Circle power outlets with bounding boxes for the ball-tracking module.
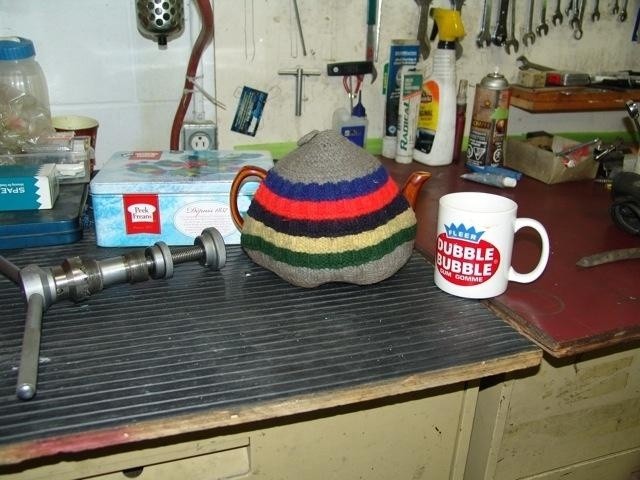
[182,120,217,151]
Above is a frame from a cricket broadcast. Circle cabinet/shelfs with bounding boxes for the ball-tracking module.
[0,169,544,480]
[373,141,640,480]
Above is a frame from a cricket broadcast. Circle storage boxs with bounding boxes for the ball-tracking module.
[87,148,276,248]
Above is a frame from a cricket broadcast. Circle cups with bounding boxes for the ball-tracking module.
[434,191,550,299]
[49,115,98,178]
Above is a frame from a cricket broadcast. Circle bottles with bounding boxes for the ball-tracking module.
[0,35,52,167]
[463,65,512,170]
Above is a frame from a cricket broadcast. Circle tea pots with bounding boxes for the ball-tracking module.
[229,130,433,290]
